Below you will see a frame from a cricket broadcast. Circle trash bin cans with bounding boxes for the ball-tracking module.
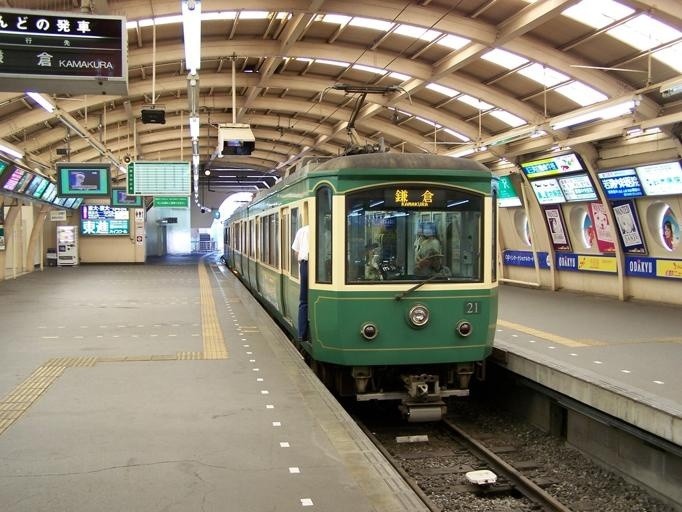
[46,248,57,267]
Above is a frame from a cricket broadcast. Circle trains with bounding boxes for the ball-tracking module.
[221,150,504,426]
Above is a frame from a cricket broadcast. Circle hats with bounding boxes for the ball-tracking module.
[426,248,444,259]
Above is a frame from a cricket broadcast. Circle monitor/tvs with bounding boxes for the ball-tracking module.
[110,187,143,208]
[56,163,112,199]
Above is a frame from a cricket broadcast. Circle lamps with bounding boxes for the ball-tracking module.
[25,91,128,174]
[441,77,682,159]
[181,1,212,214]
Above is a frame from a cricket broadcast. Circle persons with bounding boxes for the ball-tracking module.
[291,223,331,341]
[364,219,454,281]
[663,221,673,249]
